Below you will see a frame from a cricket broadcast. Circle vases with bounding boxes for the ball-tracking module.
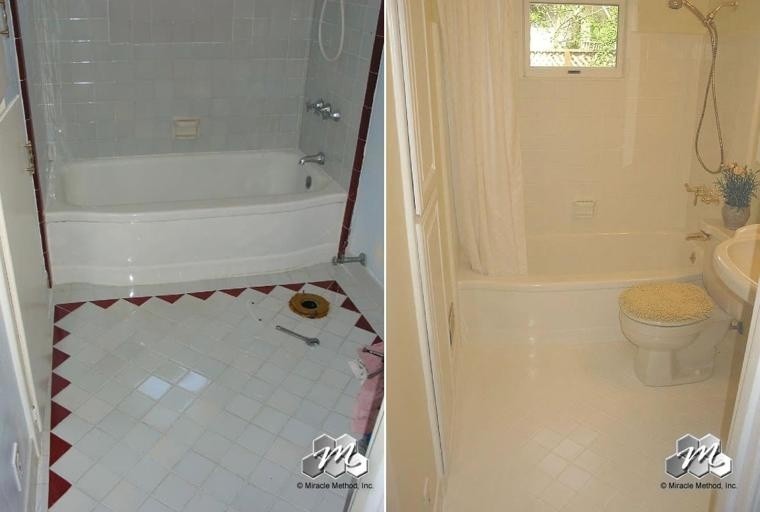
[721,201,751,230]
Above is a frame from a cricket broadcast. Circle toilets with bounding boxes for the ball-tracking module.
[618,273,745,387]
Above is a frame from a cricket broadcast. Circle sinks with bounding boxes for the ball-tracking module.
[712,224,760,305]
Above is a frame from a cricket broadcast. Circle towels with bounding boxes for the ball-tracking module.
[351,340,384,434]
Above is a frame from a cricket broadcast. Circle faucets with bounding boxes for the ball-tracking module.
[296,153,325,166]
[686,229,709,242]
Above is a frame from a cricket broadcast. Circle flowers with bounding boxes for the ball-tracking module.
[711,159,760,217]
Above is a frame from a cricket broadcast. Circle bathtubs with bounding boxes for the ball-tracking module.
[455,230,706,291]
[45,150,348,222]
